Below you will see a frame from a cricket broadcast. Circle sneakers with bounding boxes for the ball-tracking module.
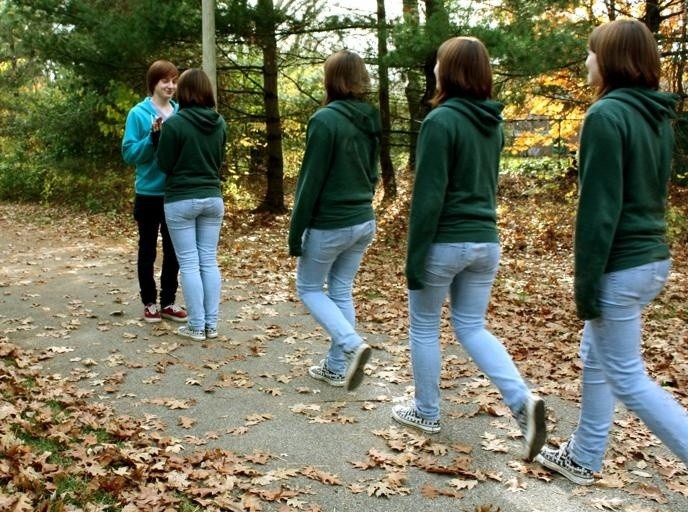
[343,344,372,391]
[514,396,546,460]
[178,326,207,340]
[308,357,346,387]
[205,327,218,338]
[144,302,162,323]
[536,442,595,486]
[393,406,442,432]
[160,304,189,322]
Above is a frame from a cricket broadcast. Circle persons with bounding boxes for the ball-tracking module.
[157,69,227,342]
[121,60,188,323]
[391,36,546,460]
[289,50,382,392]
[533,21,688,486]
